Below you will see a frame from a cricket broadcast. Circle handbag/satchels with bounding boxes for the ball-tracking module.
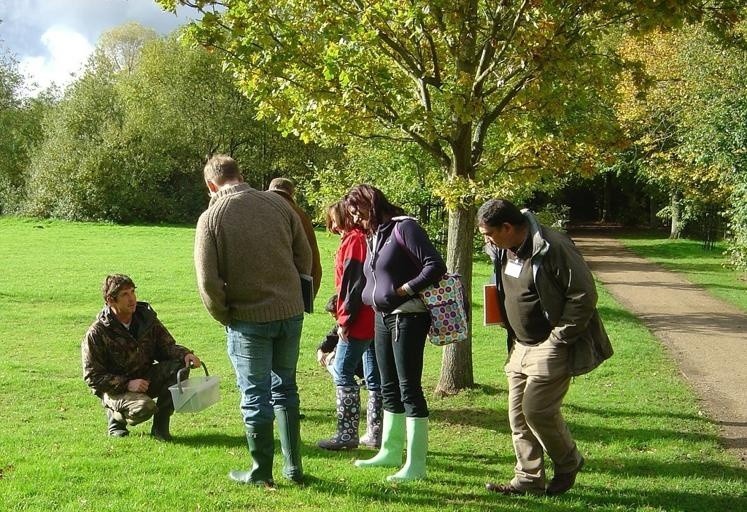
[419,267,470,347]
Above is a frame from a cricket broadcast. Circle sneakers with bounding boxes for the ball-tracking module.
[151,418,173,442]
[105,409,128,437]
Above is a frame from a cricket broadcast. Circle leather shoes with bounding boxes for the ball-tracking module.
[485,482,545,497]
[546,456,585,498]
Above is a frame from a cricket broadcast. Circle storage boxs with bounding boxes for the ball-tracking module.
[170,357,223,416]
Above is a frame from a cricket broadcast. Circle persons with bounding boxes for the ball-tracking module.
[80,271,203,444]
[263,170,323,298]
[343,183,448,481]
[191,150,316,490]
[316,293,368,391]
[475,197,617,500]
[317,200,382,452]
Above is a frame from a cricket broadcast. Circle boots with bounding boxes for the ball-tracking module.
[358,389,381,446]
[318,384,361,450]
[386,417,429,482]
[276,407,303,483]
[354,409,405,468]
[229,425,274,487]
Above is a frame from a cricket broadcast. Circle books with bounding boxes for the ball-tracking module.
[481,282,506,328]
[294,270,314,317]
